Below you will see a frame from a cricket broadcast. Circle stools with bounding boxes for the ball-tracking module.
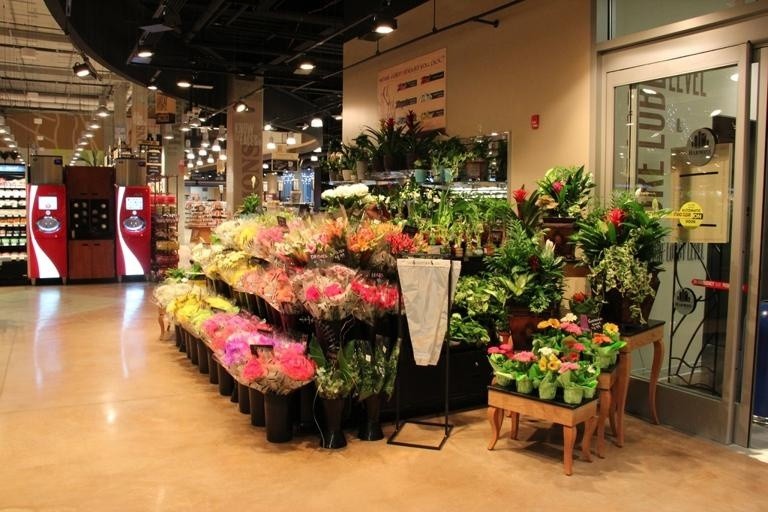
[487,318,665,476]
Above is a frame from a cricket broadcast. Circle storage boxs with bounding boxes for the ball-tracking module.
[29,155,63,184]
[115,158,147,186]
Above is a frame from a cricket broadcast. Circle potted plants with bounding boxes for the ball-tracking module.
[487,342,622,405]
[341,111,466,185]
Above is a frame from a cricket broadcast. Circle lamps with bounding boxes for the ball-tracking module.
[70,61,112,166]
[137,43,227,181]
[0,115,25,164]
[300,56,315,70]
[372,12,398,34]
[233,101,342,170]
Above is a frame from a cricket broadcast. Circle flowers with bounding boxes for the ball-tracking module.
[442,263,510,348]
[506,254,561,303]
[584,189,669,274]
[393,170,587,268]
[550,291,620,342]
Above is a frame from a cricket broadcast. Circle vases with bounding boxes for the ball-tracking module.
[594,268,659,329]
[510,301,550,350]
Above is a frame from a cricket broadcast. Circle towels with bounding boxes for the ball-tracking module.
[397,258,462,366]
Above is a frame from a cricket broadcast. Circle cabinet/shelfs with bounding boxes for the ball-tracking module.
[0,164,28,284]
[66,166,115,283]
